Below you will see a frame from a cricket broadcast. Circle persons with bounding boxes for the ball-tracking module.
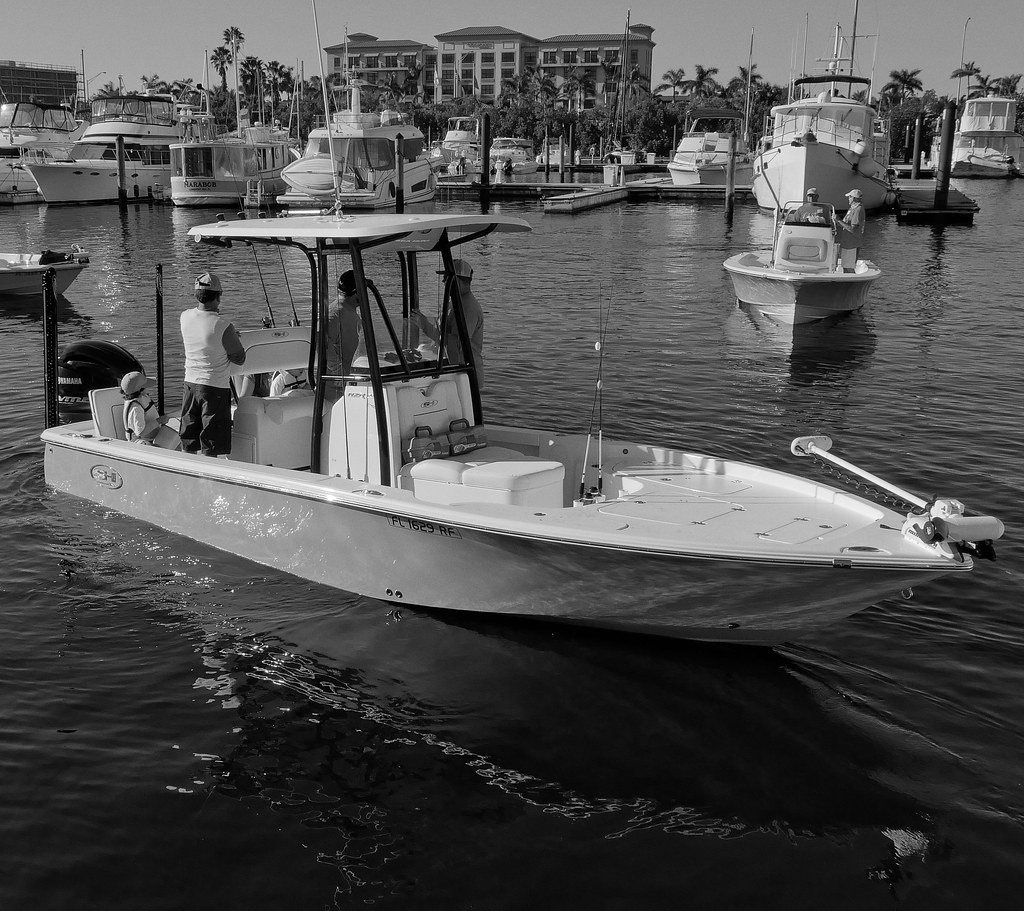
[410,259,484,394]
[121,371,181,451]
[831,189,865,269]
[793,187,830,224]
[180,273,245,457]
[316,269,365,404]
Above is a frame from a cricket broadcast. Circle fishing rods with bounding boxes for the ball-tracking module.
[207,93,277,328]
[238,94,301,326]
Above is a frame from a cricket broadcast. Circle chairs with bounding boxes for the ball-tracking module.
[87,387,127,440]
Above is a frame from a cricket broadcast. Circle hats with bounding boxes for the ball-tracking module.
[120,371,156,394]
[436,259,473,278]
[338,269,373,291]
[845,189,863,199]
[807,188,818,196]
[194,272,224,292]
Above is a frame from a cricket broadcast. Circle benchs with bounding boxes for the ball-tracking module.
[408,441,566,507]
[228,325,334,470]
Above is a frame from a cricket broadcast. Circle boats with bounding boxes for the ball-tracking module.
[721,193,882,327]
[0,243,90,296]
[1,1,1024,224]
[40,208,1006,654]
